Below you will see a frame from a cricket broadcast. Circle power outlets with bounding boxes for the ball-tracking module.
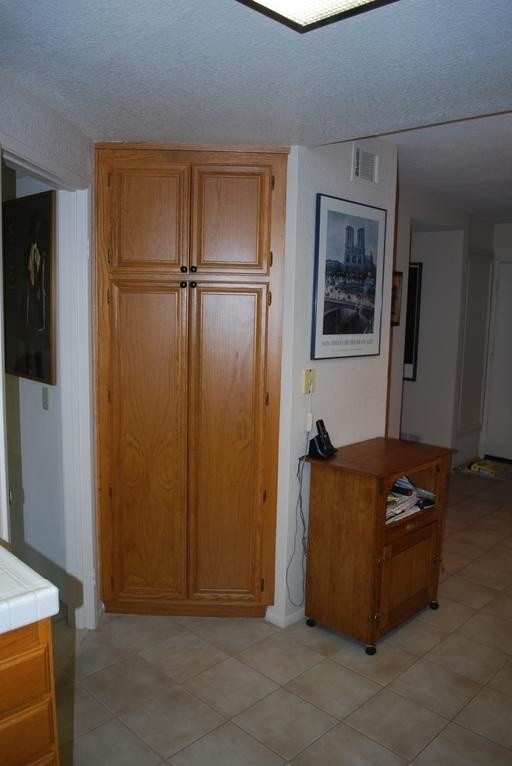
[303,367,314,393]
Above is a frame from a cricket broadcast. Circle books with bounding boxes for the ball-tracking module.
[385,476,436,526]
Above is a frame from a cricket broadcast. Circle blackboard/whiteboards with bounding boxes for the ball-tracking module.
[1,190,56,387]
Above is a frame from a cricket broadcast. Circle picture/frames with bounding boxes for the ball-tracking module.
[310,192,388,360]
[391,271,404,326]
[403,260,422,381]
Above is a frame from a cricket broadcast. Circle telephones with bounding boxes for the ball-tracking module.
[309,420,338,459]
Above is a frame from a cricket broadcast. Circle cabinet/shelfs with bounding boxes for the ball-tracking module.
[109,278,269,608]
[298,436,459,654]
[109,161,273,279]
[2,618,61,765]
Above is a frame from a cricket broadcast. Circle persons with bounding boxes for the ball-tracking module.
[27,242,48,377]
